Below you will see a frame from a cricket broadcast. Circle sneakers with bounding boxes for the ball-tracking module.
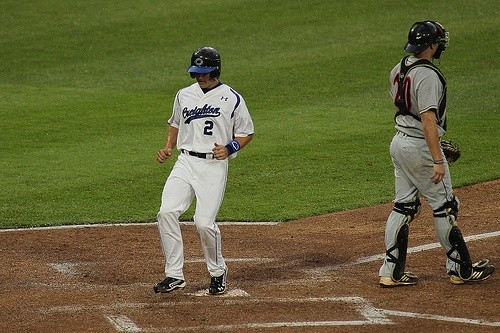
[154,276,186,293]
[210,266,228,294]
[379,270,419,285]
[450,264,496,284]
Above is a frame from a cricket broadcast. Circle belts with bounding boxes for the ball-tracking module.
[180,149,216,160]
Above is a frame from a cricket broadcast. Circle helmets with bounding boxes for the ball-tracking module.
[186,46,221,79]
[404,20,441,53]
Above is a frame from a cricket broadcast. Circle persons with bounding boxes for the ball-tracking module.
[153,47,255,295]
[377,19,495,288]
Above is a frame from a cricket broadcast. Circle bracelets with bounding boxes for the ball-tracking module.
[225,140,240,156]
[433,158,444,164]
